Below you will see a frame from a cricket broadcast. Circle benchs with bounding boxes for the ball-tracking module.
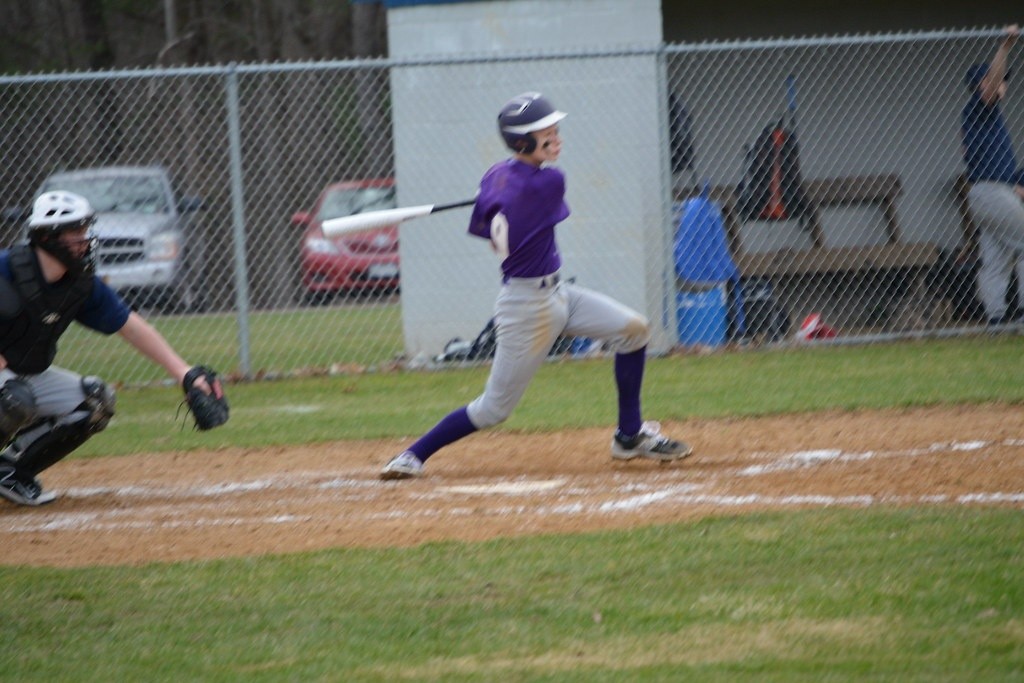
[670,171,940,329]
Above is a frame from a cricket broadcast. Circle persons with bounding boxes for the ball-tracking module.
[379,95,692,478]
[963,24,1024,326]
[0,191,222,506]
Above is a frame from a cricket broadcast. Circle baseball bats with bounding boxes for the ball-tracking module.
[321,199,476,239]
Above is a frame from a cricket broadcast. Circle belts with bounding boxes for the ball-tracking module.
[503,274,561,287]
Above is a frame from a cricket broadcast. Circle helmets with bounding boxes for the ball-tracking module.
[28,190,97,277]
[497,92,567,154]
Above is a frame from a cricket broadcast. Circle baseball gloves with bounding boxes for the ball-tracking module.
[182,365,229,431]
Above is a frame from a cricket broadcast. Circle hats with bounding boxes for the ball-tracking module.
[964,61,1011,85]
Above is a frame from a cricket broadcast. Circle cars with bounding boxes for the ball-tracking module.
[31,168,204,306]
[290,178,399,288]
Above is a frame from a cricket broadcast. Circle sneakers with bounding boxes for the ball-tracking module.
[612,421,694,460]
[0,454,56,505]
[379,453,422,478]
